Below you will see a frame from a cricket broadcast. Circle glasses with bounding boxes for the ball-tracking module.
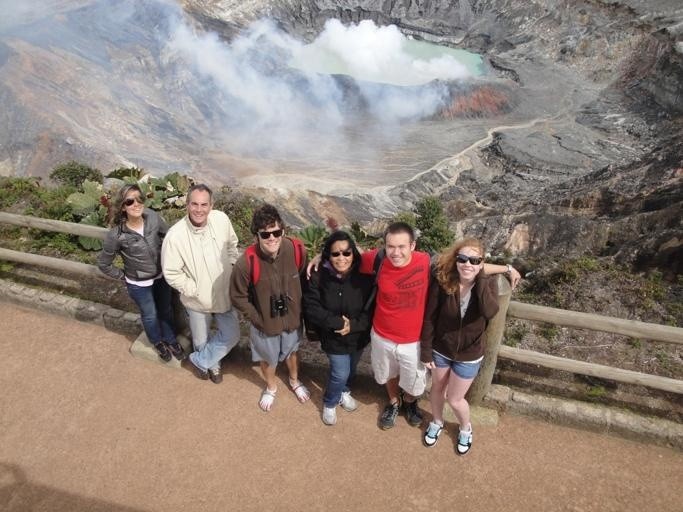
[122,195,146,206]
[329,251,353,257]
[256,229,284,239]
[455,253,484,266]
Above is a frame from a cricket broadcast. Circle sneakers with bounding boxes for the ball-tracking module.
[209,365,224,384]
[259,384,276,412]
[168,342,187,362]
[153,340,171,361]
[401,391,423,428]
[339,390,355,411]
[287,377,311,403]
[456,421,473,456]
[187,353,210,379]
[322,401,337,425]
[378,397,402,431]
[423,418,446,448]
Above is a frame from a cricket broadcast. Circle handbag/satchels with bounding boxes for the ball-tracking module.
[303,309,320,342]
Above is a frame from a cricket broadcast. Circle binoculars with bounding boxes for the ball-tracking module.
[269,292,289,318]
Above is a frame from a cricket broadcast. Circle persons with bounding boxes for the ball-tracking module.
[421,237,499,456]
[303,231,376,425]
[230,202,311,411]
[161,184,241,383]
[97,184,186,362]
[307,221,521,430]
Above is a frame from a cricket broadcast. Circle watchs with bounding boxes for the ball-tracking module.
[504,264,512,278]
